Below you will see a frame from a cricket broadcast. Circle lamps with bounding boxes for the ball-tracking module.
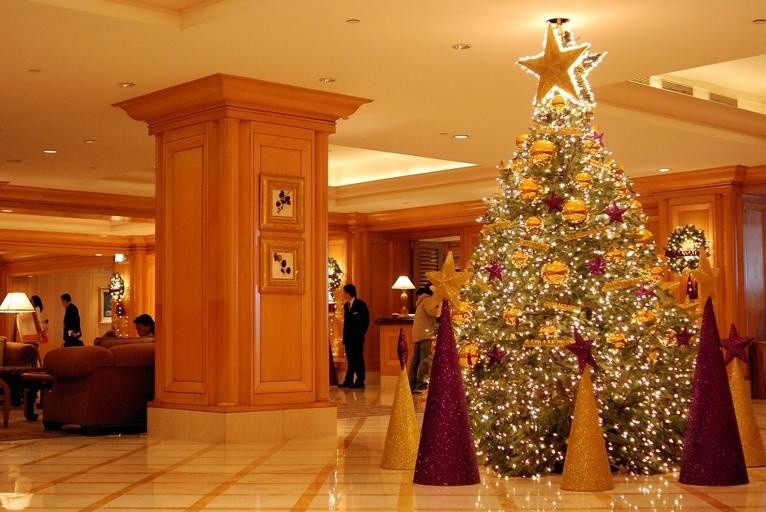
[109,272,128,318]
[0,292,36,341]
[391,275,416,316]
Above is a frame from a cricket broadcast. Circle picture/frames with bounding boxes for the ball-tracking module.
[259,173,303,231]
[98,287,113,324]
[259,235,305,294]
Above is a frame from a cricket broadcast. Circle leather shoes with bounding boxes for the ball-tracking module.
[337,383,355,388]
[349,384,365,389]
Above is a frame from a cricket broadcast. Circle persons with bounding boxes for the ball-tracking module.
[133,313,155,338]
[17,294,49,349]
[405,278,442,393]
[61,292,82,348]
[338,283,370,390]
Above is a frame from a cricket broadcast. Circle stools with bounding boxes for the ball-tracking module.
[0,379,10,427]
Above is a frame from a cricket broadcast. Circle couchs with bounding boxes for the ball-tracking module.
[0,336,44,408]
[43,336,155,434]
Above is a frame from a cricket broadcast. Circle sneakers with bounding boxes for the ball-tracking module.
[415,382,428,393]
[410,379,417,393]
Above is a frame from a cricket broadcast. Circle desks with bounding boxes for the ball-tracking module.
[376,320,414,375]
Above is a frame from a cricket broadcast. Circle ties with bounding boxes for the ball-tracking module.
[348,303,350,310]
